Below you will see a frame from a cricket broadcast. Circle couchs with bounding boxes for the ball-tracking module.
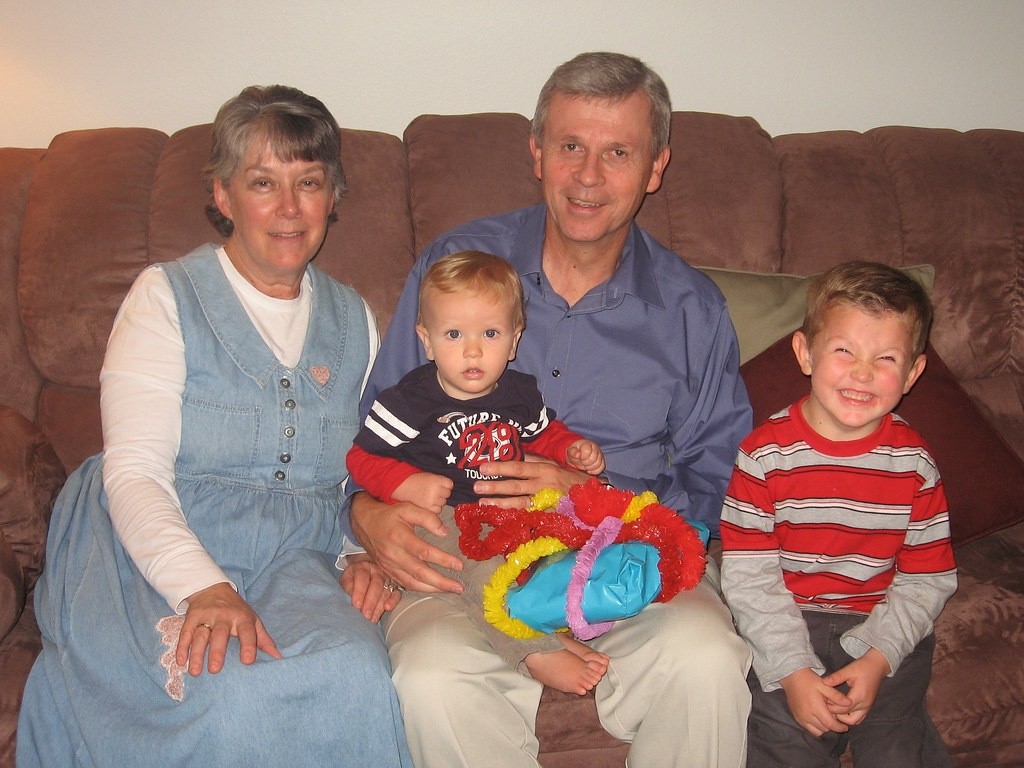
[0,111,1024,768]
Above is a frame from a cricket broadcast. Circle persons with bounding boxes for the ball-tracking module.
[14,86,413,768]
[346,251,609,696]
[719,260,958,768]
[338,51,755,768]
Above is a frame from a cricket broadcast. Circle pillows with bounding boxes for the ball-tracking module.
[742,323,1023,549]
[693,264,936,367]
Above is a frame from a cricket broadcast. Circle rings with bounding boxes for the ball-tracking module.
[530,496,533,506]
[384,585,394,592]
